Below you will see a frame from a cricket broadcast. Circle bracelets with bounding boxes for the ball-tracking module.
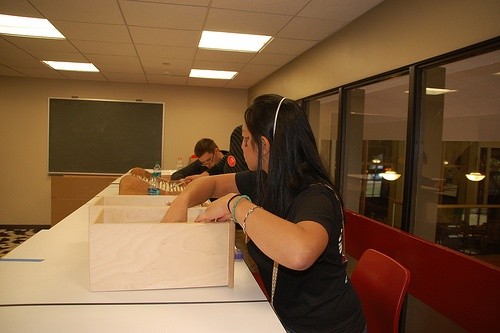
[227,194,242,212]
[242,205,263,235]
[232,195,251,223]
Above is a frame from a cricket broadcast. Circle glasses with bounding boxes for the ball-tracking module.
[199,151,214,166]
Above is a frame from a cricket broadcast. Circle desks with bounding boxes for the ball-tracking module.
[0,169,288,333]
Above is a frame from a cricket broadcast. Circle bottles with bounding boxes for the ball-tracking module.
[154,161,161,180]
[176,157,183,170]
[148,173,160,196]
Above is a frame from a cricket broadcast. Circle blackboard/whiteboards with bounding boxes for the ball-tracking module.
[46,96,165,175]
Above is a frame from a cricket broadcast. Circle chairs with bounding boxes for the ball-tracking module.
[350,248,411,333]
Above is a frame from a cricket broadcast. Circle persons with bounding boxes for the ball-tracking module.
[224,125,249,173]
[170,138,230,183]
[160,93,367,333]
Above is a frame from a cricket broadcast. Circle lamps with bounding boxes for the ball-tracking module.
[379,169,401,181]
[465,172,486,182]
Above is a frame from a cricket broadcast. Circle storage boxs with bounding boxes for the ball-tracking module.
[88,195,236,292]
